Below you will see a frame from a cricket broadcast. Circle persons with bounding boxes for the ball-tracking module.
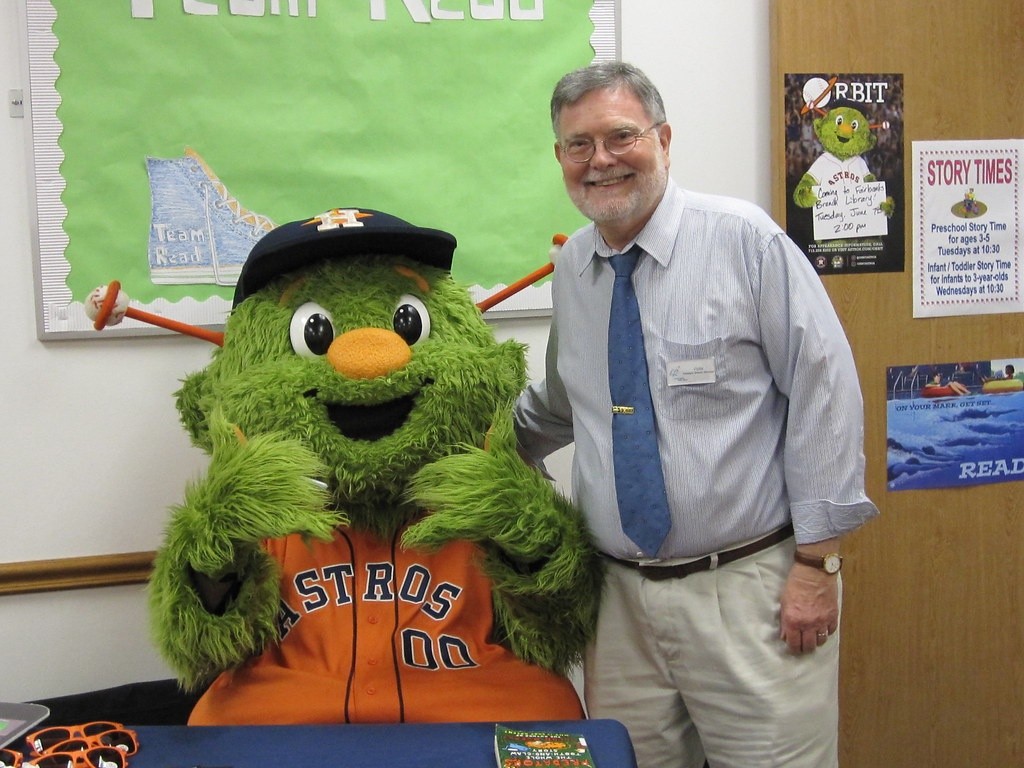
[953,363,984,385]
[513,61,879,768]
[981,364,1014,383]
[925,372,972,396]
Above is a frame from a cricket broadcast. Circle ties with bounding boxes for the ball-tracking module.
[609,246,673,559]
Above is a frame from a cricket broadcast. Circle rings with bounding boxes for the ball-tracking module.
[818,630,828,637]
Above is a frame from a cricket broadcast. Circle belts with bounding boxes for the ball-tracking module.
[598,522,795,581]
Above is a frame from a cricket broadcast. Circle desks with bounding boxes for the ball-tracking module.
[21,718,639,768]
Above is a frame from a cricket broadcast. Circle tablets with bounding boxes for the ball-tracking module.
[0,702,50,752]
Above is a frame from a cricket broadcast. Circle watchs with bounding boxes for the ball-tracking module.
[795,551,843,575]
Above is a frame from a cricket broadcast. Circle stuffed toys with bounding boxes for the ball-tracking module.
[144,208,609,726]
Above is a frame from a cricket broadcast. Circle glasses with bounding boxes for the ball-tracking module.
[0,722,138,768]
[558,121,657,162]
